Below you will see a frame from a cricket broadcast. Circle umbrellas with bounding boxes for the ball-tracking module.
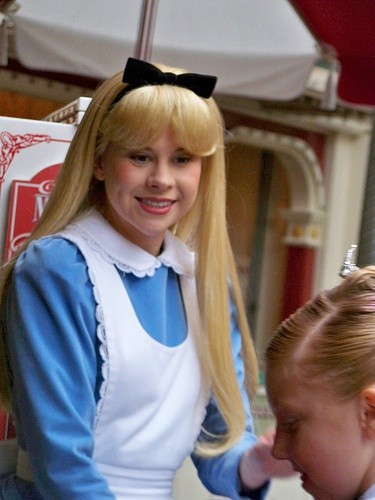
[1,0,374,115]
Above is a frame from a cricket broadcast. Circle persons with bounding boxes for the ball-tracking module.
[267,264,375,500]
[1,55,297,500]
